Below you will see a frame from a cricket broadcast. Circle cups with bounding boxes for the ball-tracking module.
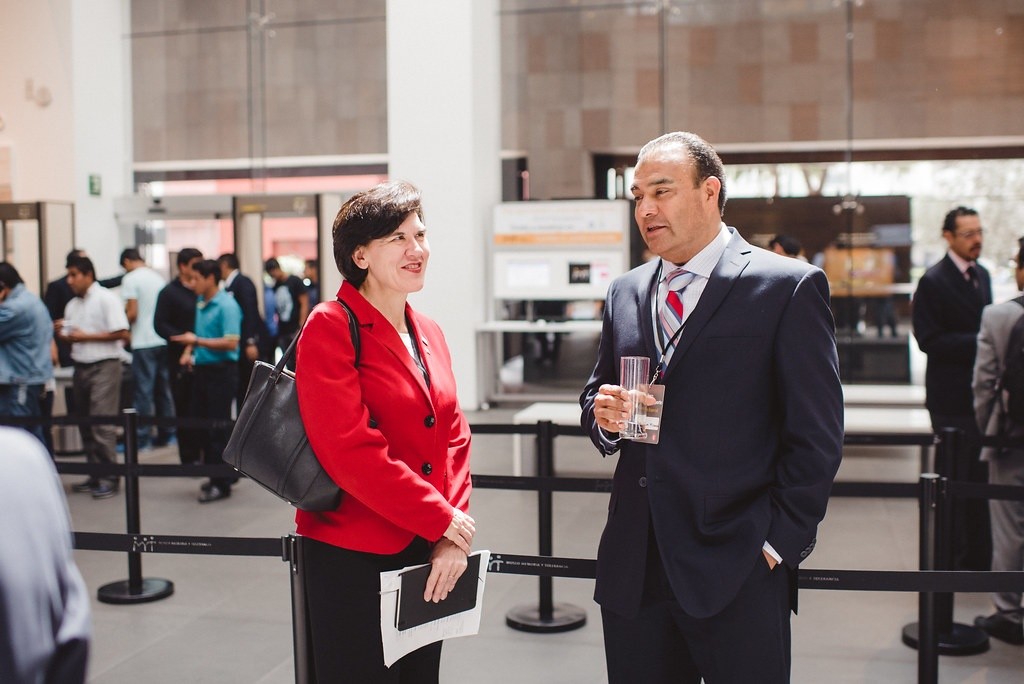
[618,355,650,440]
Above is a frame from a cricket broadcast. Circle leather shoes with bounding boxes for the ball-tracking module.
[973,612,1024,646]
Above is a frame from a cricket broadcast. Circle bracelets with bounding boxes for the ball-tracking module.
[194,337,198,346]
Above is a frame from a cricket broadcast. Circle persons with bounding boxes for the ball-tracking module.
[154,249,203,463]
[54,257,130,499]
[973,235,1024,647]
[0,427,90,684]
[219,254,318,416]
[767,235,801,260]
[43,250,124,413]
[0,261,58,459]
[578,131,845,684]
[170,260,242,503]
[294,180,476,684]
[116,248,175,453]
[911,205,993,570]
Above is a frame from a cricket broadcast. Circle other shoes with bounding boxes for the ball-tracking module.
[197,480,231,502]
[88,484,119,499]
[71,479,95,494]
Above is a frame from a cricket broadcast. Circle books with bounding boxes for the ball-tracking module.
[395,554,482,632]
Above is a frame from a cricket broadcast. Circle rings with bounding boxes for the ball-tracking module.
[448,575,455,578]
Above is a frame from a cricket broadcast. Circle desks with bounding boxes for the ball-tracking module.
[510,384,934,478]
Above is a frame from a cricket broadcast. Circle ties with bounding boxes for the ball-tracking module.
[653,266,697,386]
[964,266,981,300]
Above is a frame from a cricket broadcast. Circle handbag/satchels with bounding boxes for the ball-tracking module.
[220,299,363,515]
